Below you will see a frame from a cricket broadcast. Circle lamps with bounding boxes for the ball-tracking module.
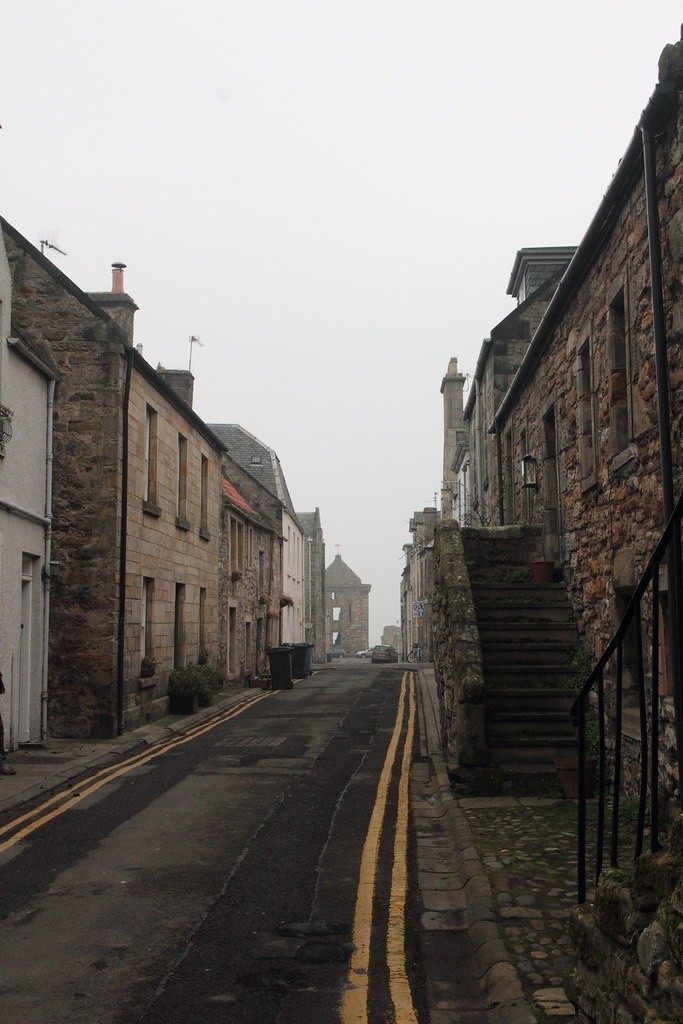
[521,454,538,490]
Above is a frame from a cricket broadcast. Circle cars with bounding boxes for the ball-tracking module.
[331,648,348,658]
[372,644,396,664]
[355,648,374,659]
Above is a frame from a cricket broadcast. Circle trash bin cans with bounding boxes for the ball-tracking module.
[282,642,307,679]
[265,646,294,690]
[307,643,315,676]
[327,653,332,662]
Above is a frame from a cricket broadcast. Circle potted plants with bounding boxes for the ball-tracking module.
[169,664,224,714]
[199,647,208,665]
[141,656,156,678]
[555,694,596,800]
[530,517,557,584]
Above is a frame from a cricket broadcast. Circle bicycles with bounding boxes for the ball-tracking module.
[407,644,426,663]
